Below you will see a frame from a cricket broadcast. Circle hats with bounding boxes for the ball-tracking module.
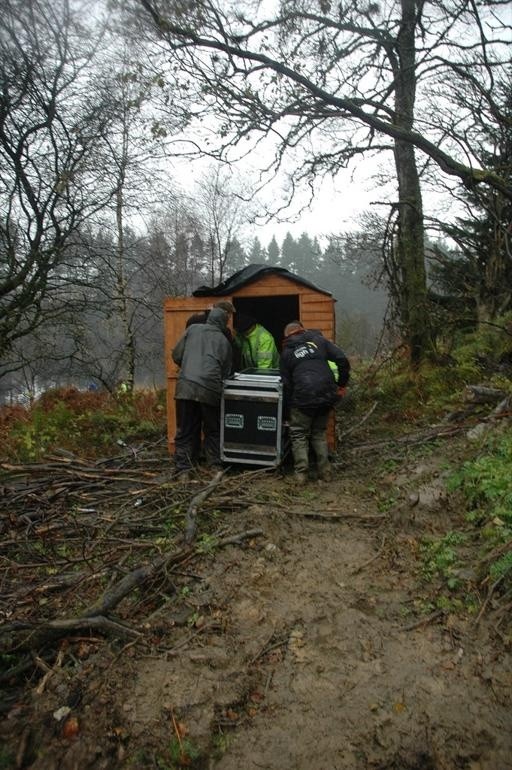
[212,299,237,314]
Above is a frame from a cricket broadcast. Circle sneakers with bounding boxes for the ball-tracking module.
[309,470,333,483]
[281,472,309,488]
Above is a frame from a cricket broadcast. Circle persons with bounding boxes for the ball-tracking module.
[171,301,351,485]
